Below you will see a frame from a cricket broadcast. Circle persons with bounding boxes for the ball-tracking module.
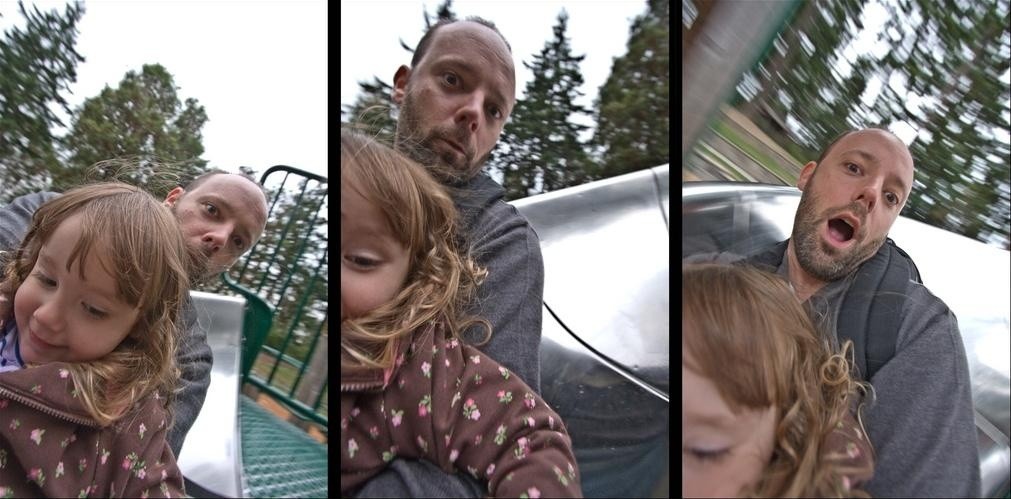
[0,166,270,464]
[682,123,988,498]
[680,247,878,499]
[0,176,198,499]
[341,14,548,496]
[339,126,584,498]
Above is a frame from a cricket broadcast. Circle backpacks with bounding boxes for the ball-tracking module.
[736,236,924,380]
